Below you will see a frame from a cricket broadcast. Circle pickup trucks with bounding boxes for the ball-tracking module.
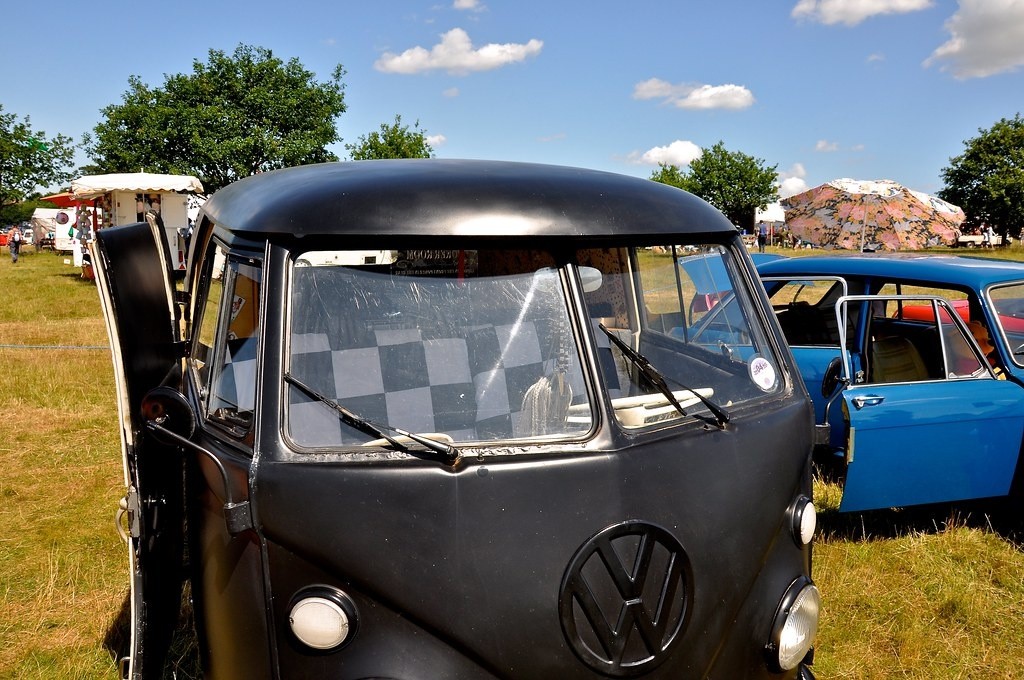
[956,222,1013,250]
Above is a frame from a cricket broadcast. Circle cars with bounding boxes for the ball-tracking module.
[87,154,849,678]
[665,250,1024,549]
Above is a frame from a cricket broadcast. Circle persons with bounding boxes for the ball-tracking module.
[776,226,805,250]
[978,222,1011,250]
[1019,227,1024,245]
[757,219,768,253]
[6,223,22,263]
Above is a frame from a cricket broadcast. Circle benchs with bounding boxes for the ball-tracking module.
[777,305,857,351]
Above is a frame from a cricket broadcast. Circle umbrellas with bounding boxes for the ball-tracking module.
[40,192,102,206]
[779,178,966,253]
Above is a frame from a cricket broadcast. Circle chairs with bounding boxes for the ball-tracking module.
[872,337,928,382]
[221,303,624,446]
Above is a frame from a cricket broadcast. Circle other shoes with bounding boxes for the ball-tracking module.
[13,259,17,263]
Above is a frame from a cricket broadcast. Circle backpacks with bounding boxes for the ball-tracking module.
[12,229,19,242]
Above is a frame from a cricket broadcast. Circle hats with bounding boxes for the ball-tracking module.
[13,223,16,226]
